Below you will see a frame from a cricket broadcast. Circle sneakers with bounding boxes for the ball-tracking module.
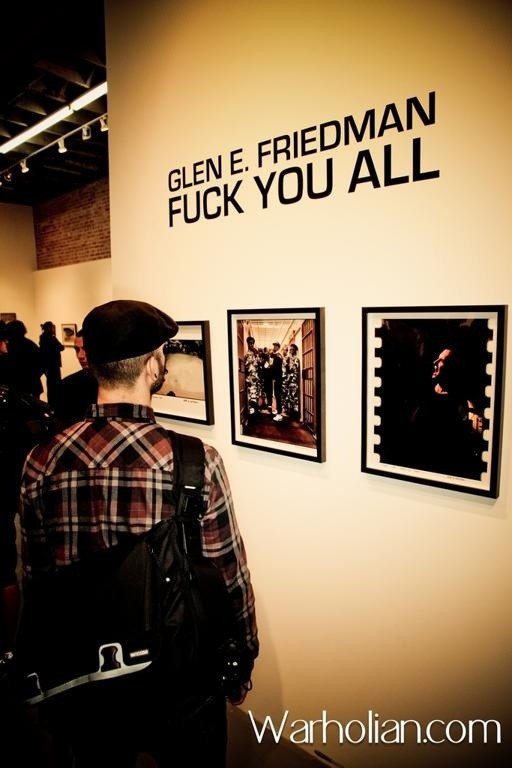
[261,409,288,421]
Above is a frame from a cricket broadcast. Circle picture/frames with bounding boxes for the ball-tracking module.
[61,323,78,347]
[152,320,215,425]
[226,305,509,499]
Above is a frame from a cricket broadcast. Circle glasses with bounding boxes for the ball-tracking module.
[249,342,254,344]
[273,342,280,347]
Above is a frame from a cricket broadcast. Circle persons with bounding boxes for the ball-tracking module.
[244,336,300,423]
[0,299,260,768]
[375,319,484,480]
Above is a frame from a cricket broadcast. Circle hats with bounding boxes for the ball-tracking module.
[290,343,298,350]
[81,300,179,365]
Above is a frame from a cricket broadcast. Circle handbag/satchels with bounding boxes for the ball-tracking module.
[8,430,233,753]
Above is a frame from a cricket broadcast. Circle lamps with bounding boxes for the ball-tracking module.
[0,111,108,175]
